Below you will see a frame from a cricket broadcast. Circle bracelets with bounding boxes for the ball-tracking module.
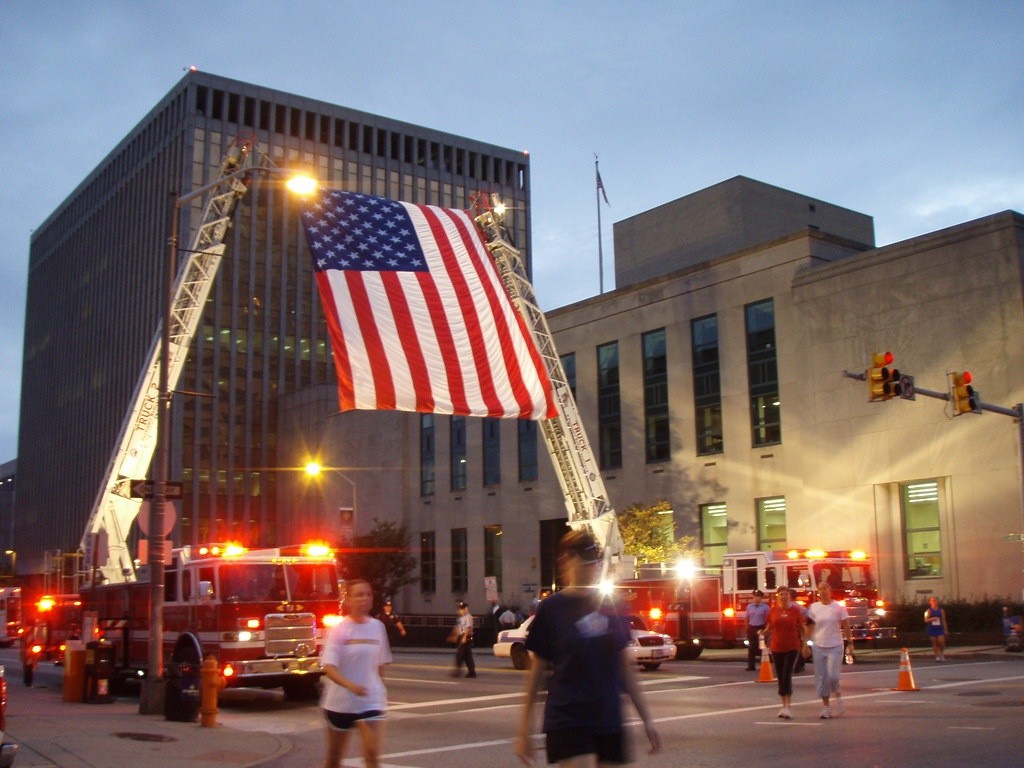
[848,641,852,644]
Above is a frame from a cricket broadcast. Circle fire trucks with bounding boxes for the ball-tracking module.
[18,128,352,704]
[470,191,900,663]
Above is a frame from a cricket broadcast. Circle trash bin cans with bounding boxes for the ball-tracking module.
[83,641,116,703]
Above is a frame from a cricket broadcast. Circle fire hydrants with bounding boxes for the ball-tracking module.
[197,653,227,727]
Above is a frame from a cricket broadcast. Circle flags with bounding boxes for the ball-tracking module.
[595,161,612,207]
[281,174,560,420]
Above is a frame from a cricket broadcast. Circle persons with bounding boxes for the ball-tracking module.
[745,585,855,720]
[375,601,406,647]
[924,596,949,662]
[447,602,477,678]
[513,529,664,768]
[498,605,524,630]
[319,576,392,768]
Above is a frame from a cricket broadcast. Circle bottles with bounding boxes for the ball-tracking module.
[845,647,854,664]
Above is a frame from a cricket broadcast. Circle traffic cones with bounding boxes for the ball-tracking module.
[755,648,780,682]
[891,647,920,690]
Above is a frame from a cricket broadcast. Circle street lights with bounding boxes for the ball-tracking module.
[305,461,358,540]
[140,166,319,716]
[4,550,17,577]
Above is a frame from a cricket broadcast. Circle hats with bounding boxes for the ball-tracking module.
[753,590,764,598]
[791,589,796,596]
[457,602,468,608]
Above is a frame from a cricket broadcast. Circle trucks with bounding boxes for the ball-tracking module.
[0,586,23,647]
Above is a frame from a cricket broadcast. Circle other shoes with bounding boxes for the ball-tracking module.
[941,658,945,661]
[448,671,462,677]
[784,711,792,718]
[936,657,941,661]
[465,672,476,678]
[778,708,784,718]
[745,667,755,671]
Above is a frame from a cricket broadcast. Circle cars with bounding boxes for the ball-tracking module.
[493,614,679,671]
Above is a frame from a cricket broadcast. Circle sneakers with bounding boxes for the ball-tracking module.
[836,703,846,716]
[821,706,832,718]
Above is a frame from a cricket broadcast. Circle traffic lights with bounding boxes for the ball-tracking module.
[950,371,976,416]
[867,351,902,403]
